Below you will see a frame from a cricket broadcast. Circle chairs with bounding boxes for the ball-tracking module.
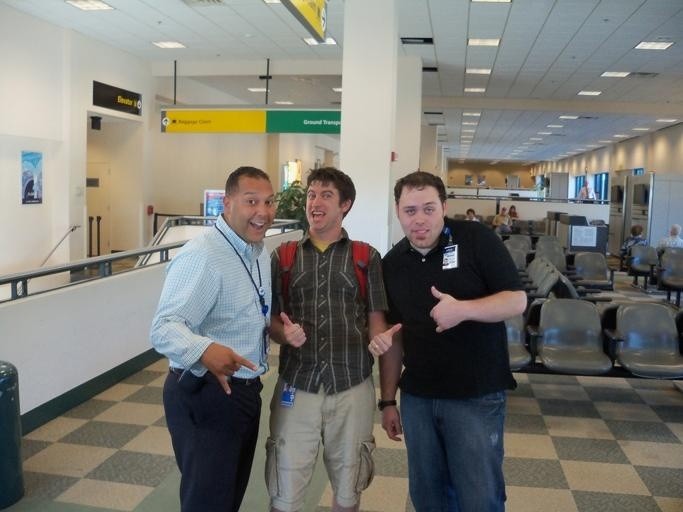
[451,213,683,383]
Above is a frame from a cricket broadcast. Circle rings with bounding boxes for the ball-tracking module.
[437,291,443,299]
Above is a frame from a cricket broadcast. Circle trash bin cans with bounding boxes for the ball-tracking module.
[0,361,24,510]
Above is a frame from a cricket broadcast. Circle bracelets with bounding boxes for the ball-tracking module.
[377,398,399,411]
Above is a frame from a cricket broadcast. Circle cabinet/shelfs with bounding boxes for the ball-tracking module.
[606,176,628,257]
[625,173,683,246]
[543,171,569,202]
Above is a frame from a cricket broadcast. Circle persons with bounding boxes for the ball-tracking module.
[461,174,683,266]
[146,165,275,512]
[376,171,529,512]
[270,166,402,512]
